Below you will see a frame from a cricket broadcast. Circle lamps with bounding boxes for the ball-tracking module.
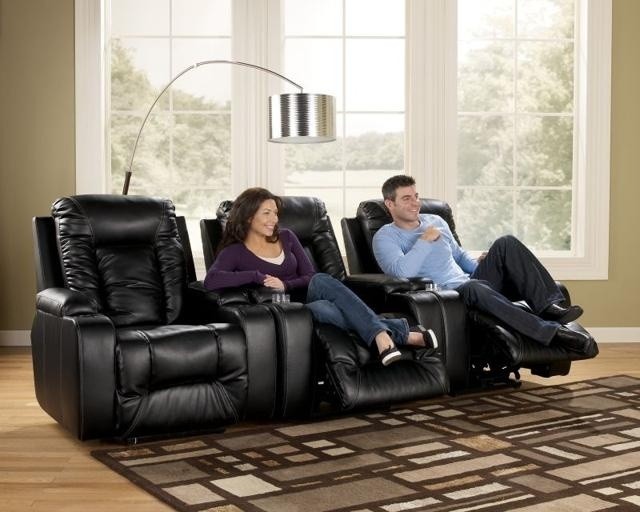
[121,59,337,197]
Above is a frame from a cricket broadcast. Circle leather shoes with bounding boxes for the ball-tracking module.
[551,326,591,354]
[537,302,584,326]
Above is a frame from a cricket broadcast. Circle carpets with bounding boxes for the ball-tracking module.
[89,375,639,512]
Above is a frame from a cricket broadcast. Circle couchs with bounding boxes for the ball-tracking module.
[31,194,600,440]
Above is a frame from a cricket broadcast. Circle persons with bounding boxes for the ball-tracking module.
[371,174,591,354]
[202,187,440,368]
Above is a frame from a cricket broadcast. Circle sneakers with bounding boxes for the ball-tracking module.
[379,344,403,367]
[409,324,439,350]
